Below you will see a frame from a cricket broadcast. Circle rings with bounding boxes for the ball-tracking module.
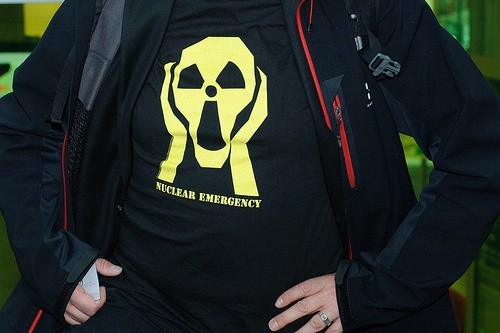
[318,311,332,327]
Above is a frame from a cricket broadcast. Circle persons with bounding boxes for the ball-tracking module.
[0,0,500,333]
